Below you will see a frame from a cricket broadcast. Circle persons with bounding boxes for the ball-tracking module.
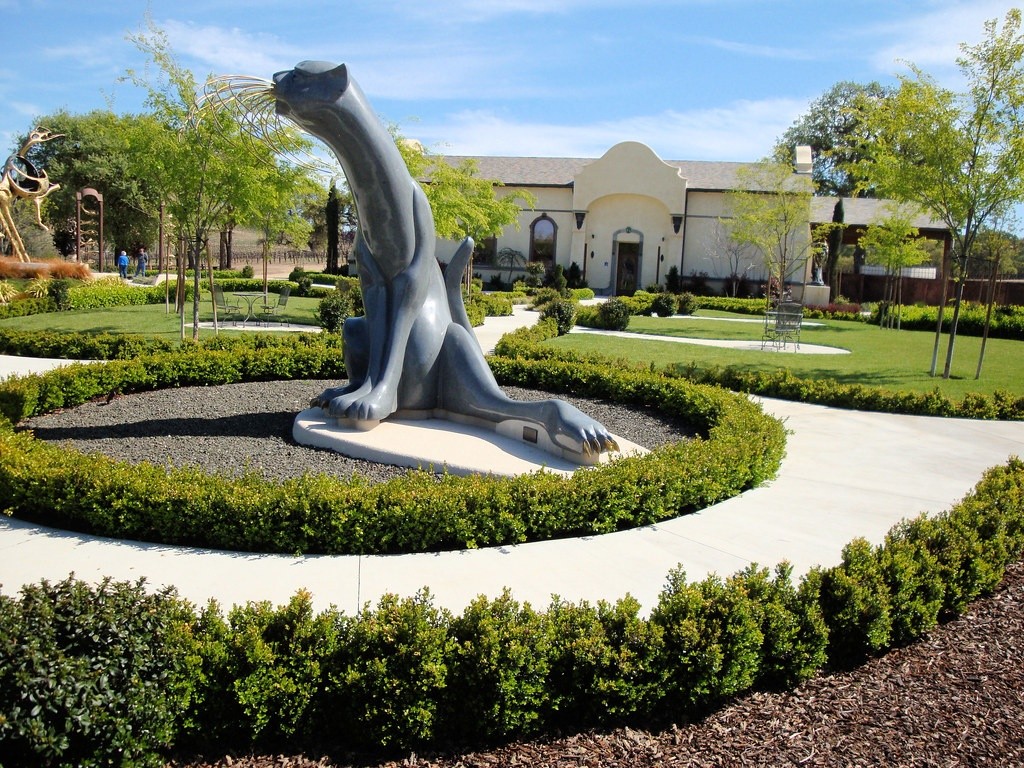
[118,251,129,278]
[1001,294,1009,314]
[770,277,793,308]
[624,253,636,287]
[133,249,148,277]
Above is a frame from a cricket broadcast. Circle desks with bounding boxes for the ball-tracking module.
[233,293,267,326]
[762,311,803,349]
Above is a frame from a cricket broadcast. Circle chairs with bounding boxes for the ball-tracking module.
[258,285,291,327]
[212,284,245,328]
[774,302,801,352]
